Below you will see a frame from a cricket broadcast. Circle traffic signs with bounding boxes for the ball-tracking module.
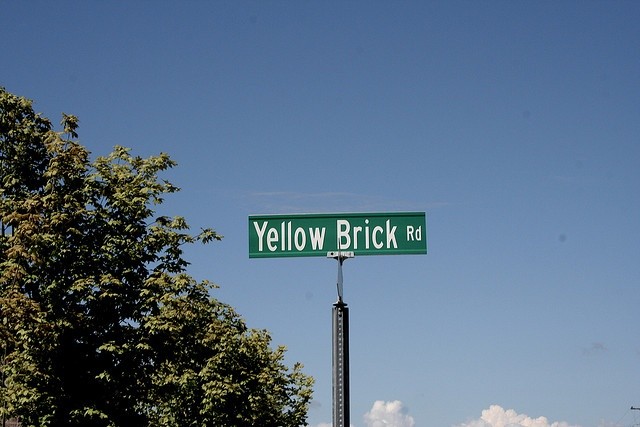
[246,210,428,258]
